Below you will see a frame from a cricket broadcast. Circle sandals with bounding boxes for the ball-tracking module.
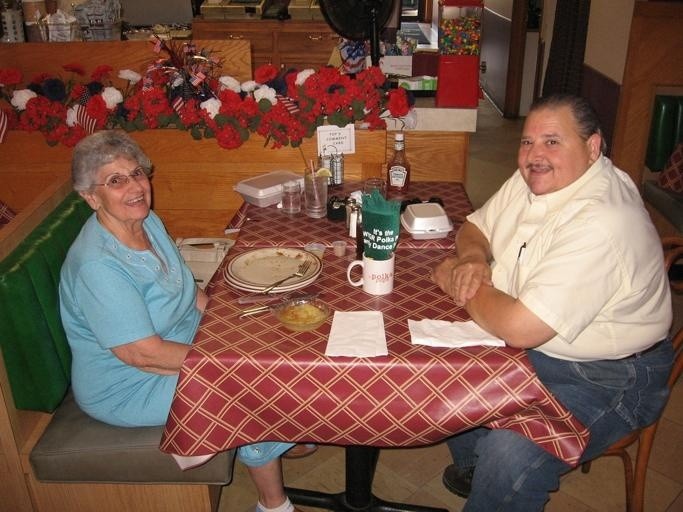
[280,444,319,459]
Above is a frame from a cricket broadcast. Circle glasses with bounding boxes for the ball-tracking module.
[92,168,150,189]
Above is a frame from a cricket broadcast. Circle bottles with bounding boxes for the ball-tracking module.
[320,151,344,186]
[386,134,411,199]
[278,182,302,215]
[343,197,362,239]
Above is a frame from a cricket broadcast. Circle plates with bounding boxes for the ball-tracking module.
[221,246,322,293]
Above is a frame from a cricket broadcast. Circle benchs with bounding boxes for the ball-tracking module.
[0,171,237,512]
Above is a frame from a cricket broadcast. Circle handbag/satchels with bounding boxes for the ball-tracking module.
[173,236,236,288]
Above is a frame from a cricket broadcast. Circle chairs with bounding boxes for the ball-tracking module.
[574,236,682,512]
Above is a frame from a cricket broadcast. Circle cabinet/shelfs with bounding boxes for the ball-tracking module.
[191,14,343,69]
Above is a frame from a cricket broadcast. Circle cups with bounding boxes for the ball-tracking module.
[301,167,327,218]
[304,244,325,261]
[345,252,397,296]
[332,241,346,257]
[363,177,384,197]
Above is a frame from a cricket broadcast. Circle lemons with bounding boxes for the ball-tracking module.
[317,168,332,177]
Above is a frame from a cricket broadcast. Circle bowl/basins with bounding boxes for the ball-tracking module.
[270,298,330,332]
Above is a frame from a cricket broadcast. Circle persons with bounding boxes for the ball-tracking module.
[429,92,676,510]
[56,129,316,511]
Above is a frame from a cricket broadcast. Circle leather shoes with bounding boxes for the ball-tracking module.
[443,463,471,499]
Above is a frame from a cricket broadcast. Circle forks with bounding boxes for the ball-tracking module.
[255,258,314,294]
